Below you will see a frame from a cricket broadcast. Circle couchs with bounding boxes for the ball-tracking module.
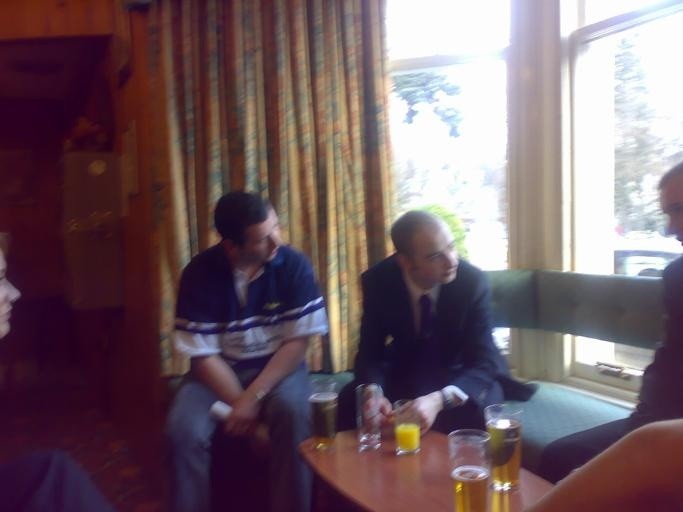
[484,271,662,475]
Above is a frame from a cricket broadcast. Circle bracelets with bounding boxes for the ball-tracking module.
[437,388,457,410]
[246,380,267,403]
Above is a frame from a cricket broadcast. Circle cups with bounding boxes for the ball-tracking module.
[309,375,421,456]
[448,402,523,511]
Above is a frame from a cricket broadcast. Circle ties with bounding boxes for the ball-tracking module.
[418,297,431,346]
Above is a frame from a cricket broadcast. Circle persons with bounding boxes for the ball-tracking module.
[0,232,115,512]
[522,159,683,485]
[161,191,329,511]
[334,205,541,445]
